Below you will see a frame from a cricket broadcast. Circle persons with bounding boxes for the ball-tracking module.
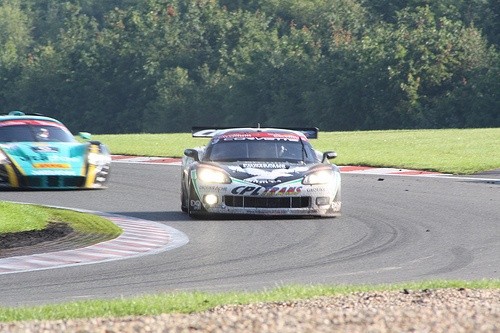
[33,127,50,140]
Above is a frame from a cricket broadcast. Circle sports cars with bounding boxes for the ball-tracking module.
[180,128,343,219]
[0,111,113,190]
